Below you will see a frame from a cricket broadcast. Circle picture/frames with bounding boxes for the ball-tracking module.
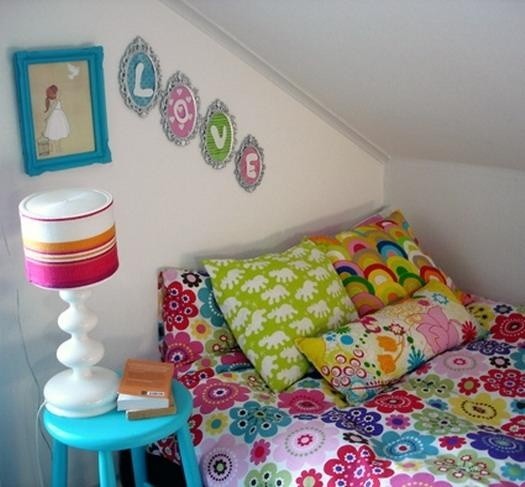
[12,45,113,176]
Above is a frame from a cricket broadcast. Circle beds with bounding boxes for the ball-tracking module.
[143,250,524,486]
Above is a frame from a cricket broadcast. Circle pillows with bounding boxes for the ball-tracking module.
[158,263,237,370]
[296,277,483,405]
[204,241,359,390]
[308,211,476,314]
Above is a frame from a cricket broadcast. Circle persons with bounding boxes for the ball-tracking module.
[42,85,71,152]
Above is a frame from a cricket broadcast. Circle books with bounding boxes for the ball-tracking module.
[116,358,175,412]
[126,391,176,421]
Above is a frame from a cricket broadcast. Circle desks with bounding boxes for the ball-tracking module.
[40,367,203,485]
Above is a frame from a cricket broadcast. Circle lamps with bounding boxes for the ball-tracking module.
[16,184,120,418]
